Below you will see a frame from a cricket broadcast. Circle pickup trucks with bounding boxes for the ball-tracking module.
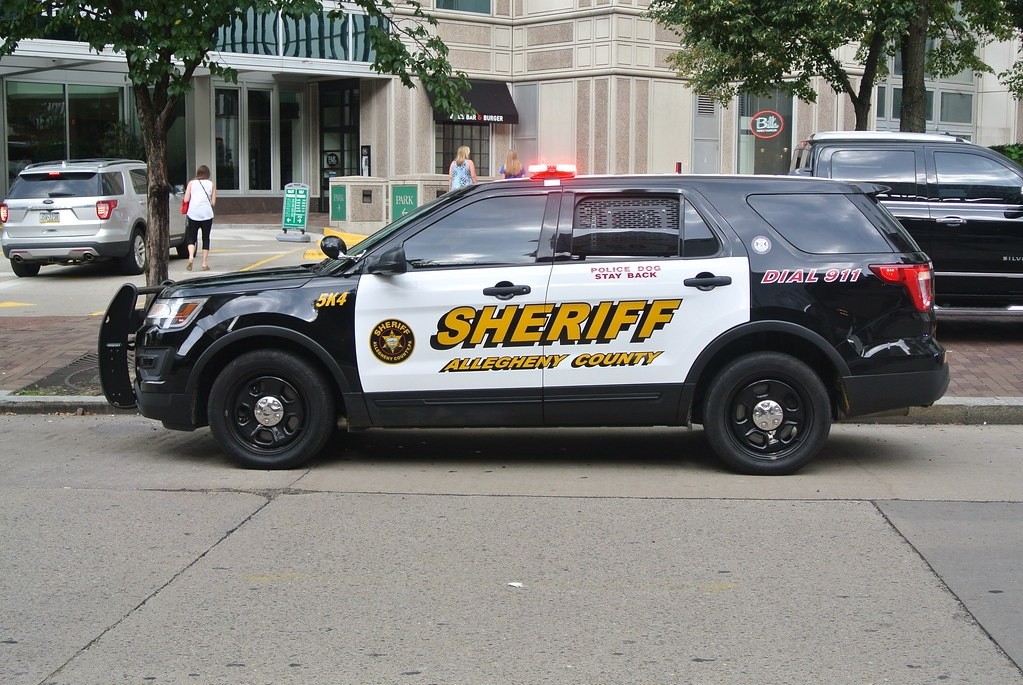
[784,130,1023,323]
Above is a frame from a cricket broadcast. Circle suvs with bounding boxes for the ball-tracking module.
[1,157,199,277]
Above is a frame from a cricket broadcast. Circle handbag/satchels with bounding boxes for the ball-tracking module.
[180,198,189,215]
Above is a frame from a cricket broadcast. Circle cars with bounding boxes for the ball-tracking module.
[98,165,950,476]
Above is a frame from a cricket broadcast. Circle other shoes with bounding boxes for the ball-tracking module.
[202,266,211,271]
[187,262,193,271]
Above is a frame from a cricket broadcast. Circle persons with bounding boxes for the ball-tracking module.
[184,165,216,271]
[448,146,477,192]
[499,149,525,179]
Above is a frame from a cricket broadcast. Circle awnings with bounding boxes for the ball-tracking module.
[433,77,519,124]
